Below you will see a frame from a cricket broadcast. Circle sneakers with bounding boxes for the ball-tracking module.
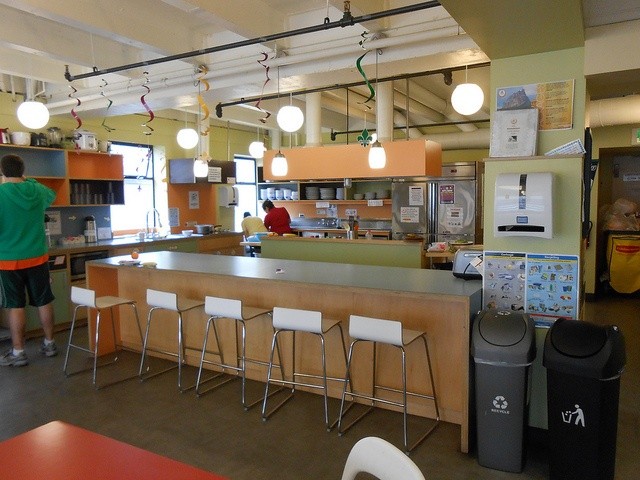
[0,349,28,367]
[38,342,57,356]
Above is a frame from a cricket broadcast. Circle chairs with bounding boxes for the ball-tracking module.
[336,313,441,456]
[194,294,285,412]
[62,284,150,391]
[261,306,355,433]
[340,436,426,480]
[136,288,225,394]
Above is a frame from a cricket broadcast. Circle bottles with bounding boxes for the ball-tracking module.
[365,229,374,239]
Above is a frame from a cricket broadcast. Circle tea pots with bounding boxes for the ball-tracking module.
[336,217,342,229]
[1,128,11,144]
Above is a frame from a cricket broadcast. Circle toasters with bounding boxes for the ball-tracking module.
[451,248,483,280]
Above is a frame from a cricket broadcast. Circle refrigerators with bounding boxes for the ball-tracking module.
[391,161,479,247]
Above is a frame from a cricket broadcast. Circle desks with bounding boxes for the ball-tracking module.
[0,418,241,480]
[424,244,483,268]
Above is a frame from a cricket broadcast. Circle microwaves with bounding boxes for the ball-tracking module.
[70,251,109,281]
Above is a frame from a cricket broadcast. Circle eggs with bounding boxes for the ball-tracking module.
[131,251,138,260]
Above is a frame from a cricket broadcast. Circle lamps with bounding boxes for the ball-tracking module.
[194,63,209,178]
[16,78,50,130]
[451,65,484,116]
[177,112,198,151]
[368,48,387,170]
[276,92,304,133]
[248,127,267,159]
[270,66,288,177]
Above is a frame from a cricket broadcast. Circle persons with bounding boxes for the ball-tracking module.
[241,212,267,238]
[262,199,291,236]
[0,154,58,367]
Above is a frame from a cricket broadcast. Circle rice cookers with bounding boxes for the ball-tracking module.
[70,129,100,151]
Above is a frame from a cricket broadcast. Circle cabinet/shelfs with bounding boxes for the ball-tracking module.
[253,180,394,239]
[144,239,198,254]
[198,231,247,257]
[0,142,128,209]
[16,251,74,334]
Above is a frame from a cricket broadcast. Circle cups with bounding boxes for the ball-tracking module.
[106,180,116,203]
[93,193,103,204]
[80,182,92,205]
[71,181,79,204]
[138,232,146,240]
[346,230,359,240]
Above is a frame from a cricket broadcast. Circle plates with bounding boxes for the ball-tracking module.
[336,187,344,200]
[320,187,336,200]
[118,260,141,266]
[353,193,364,200]
[365,192,376,199]
[283,233,296,237]
[376,190,391,199]
[304,186,319,200]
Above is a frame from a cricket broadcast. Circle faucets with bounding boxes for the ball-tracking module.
[145,208,162,239]
[330,219,336,225]
[318,218,325,226]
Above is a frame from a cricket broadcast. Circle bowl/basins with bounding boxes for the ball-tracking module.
[291,191,299,200]
[284,189,292,200]
[431,241,448,249]
[451,242,474,251]
[260,188,268,201]
[182,230,194,236]
[267,187,276,201]
[275,189,284,200]
[142,262,158,269]
[254,232,268,242]
[11,131,33,146]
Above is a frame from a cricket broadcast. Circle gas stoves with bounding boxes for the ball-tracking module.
[192,232,218,237]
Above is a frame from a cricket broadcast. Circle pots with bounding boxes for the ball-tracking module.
[33,133,48,147]
[194,224,223,234]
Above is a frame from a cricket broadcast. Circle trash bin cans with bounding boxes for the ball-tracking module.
[542,317,627,480]
[471,309,536,473]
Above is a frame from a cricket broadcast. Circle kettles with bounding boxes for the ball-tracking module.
[84,215,97,243]
[46,126,65,148]
